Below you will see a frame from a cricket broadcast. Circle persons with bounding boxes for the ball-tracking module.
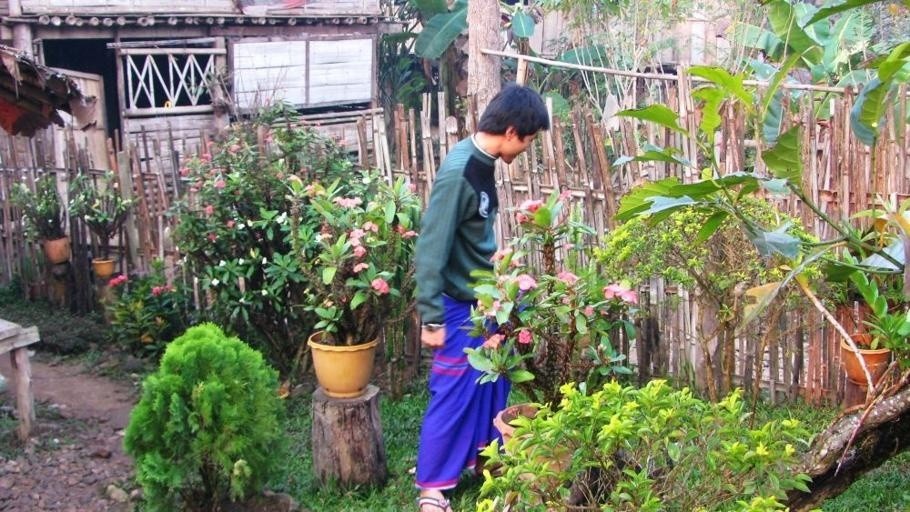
[408,87,550,511]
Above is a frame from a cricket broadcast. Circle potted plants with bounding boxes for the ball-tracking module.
[63,170,136,279]
[15,167,72,268]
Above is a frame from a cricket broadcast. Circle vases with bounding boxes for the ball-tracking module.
[493,402,567,464]
[841,331,894,388]
[304,327,384,400]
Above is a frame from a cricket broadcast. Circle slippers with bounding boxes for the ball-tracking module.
[415,488,453,512]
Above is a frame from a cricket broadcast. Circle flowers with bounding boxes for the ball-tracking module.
[457,190,642,413]
[282,170,427,344]
